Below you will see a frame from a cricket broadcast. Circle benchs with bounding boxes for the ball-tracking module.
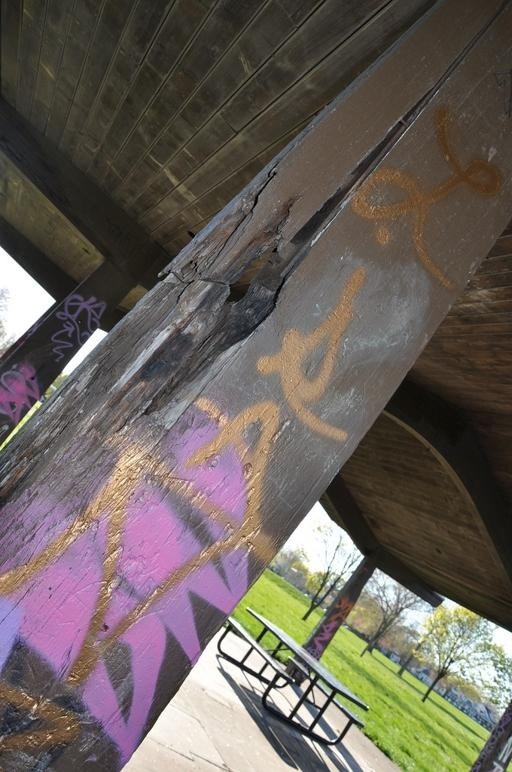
[228,616,295,685]
[288,655,366,730]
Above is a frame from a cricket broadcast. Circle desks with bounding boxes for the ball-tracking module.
[246,605,370,746]
